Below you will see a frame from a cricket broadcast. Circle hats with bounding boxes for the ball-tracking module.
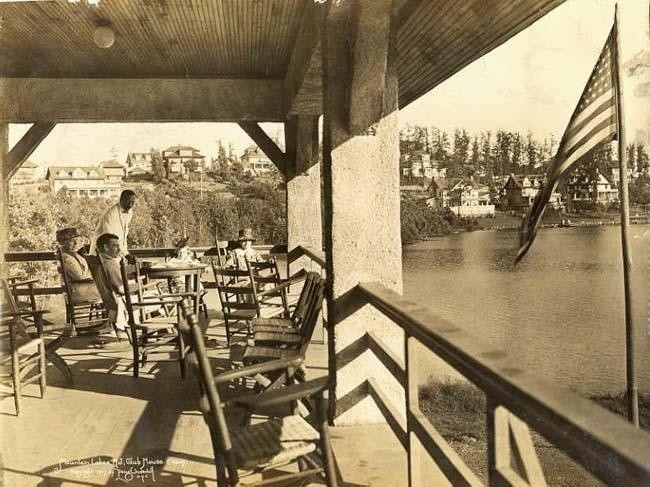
[55,228,80,242]
[236,229,256,241]
[173,236,190,253]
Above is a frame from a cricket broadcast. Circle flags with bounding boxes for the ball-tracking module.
[514,23,619,262]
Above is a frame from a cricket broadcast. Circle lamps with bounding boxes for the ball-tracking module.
[89,14,118,51]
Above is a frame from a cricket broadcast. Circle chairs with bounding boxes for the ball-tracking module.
[0,240,341,487]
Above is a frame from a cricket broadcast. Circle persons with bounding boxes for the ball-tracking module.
[168,237,204,294]
[90,190,136,265]
[56,227,101,304]
[224,228,265,304]
[96,233,174,335]
